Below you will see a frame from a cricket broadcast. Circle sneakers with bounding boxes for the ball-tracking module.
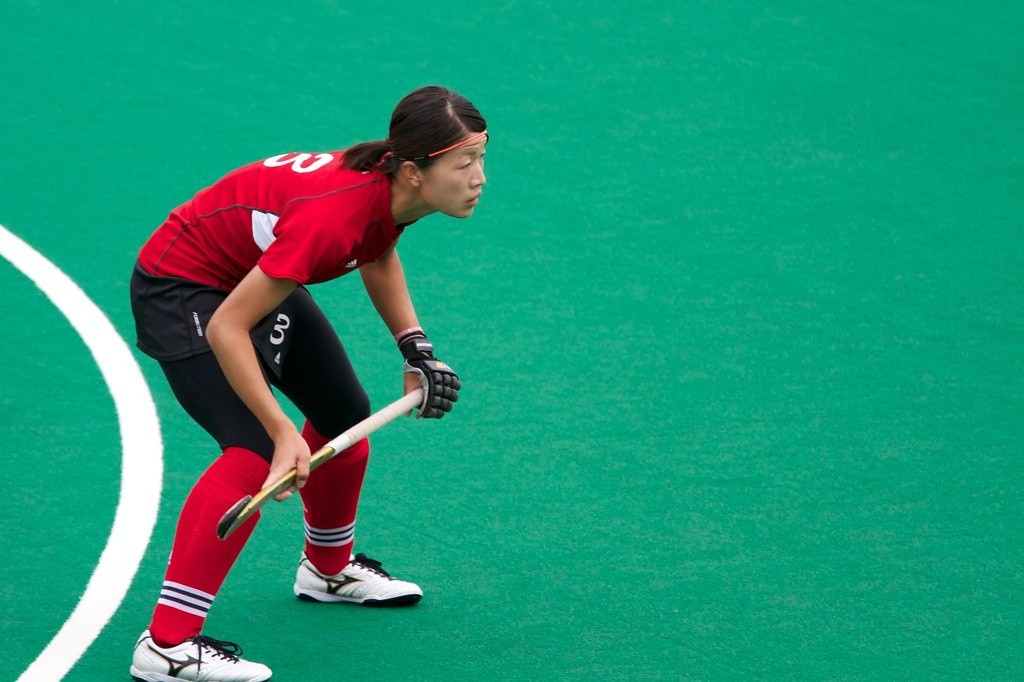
[293,550,423,607]
[129,630,272,682]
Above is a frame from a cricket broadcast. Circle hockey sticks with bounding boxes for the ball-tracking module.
[215,386,427,543]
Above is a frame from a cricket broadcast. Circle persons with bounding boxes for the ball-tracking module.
[123,84,489,682]
